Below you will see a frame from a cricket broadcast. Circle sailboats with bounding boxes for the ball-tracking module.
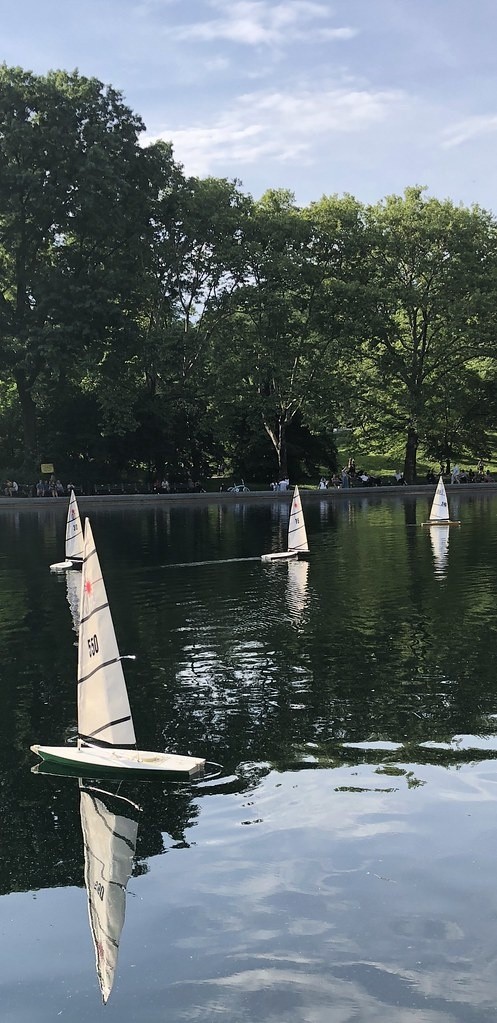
[29,517,204,782]
[50,490,84,572]
[420,475,461,525]
[27,763,144,1004]
[261,484,310,562]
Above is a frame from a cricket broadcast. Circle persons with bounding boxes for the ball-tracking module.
[317,458,492,489]
[4,474,289,497]
